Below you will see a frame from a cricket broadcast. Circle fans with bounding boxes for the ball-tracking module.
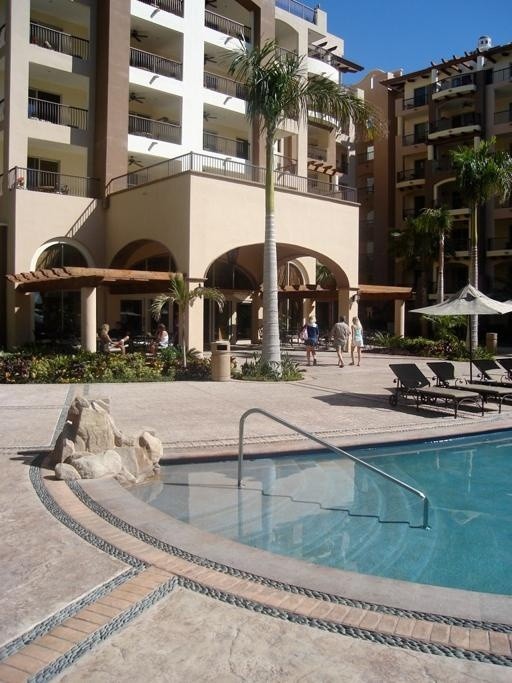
[205,0,217,9]
[129,93,145,104]
[204,54,218,65]
[131,30,149,42]
[204,112,216,122]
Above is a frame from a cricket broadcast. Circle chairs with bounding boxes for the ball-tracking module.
[387,357,512,420]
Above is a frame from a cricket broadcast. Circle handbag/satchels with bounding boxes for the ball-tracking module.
[299,324,309,341]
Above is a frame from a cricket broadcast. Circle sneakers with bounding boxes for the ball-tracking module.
[306,359,317,366]
[336,361,360,368]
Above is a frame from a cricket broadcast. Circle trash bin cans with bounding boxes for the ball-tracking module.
[486,332,497,352]
[212,340,229,380]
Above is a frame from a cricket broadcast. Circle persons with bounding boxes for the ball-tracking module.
[302,314,320,365]
[150,323,169,353]
[350,316,363,366]
[100,323,129,356]
[331,316,349,367]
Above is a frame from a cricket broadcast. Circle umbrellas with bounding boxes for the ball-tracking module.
[412,283,512,384]
